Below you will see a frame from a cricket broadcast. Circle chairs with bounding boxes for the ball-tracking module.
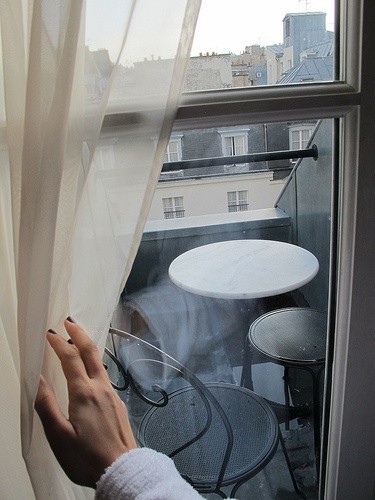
[240,309,334,495]
[65,327,306,500]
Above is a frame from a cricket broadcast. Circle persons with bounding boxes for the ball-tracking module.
[112,212,257,401]
[24,317,208,500]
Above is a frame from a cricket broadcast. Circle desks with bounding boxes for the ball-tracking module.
[169,240,319,425]
